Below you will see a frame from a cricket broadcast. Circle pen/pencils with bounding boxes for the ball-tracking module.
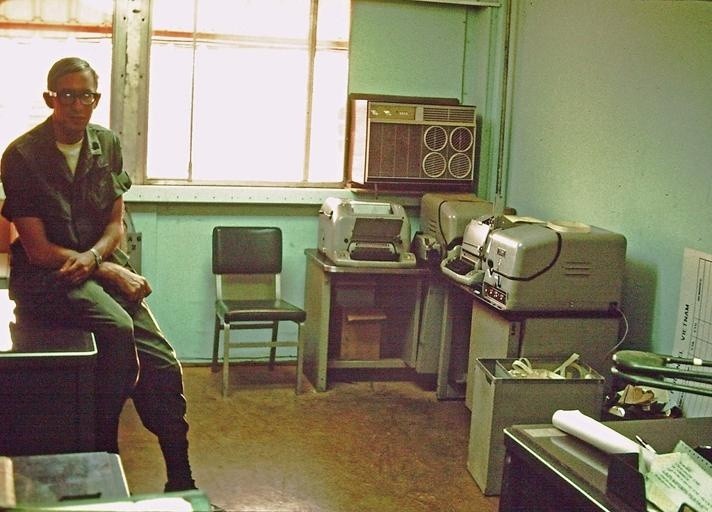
[635,435,657,456]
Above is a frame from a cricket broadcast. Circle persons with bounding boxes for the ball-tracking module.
[0,58,228,512]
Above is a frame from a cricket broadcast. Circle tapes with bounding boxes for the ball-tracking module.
[547,219,593,235]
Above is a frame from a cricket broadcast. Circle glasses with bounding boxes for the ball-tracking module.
[48,89,101,107]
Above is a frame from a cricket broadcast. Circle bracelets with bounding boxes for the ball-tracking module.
[89,247,103,265]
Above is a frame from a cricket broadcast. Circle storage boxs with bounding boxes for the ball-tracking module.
[336,308,387,360]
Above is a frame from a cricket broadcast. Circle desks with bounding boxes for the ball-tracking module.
[498,416,712,512]
[0,277,131,512]
[304,248,621,411]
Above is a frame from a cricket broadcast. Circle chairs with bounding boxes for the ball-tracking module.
[210,225,307,397]
